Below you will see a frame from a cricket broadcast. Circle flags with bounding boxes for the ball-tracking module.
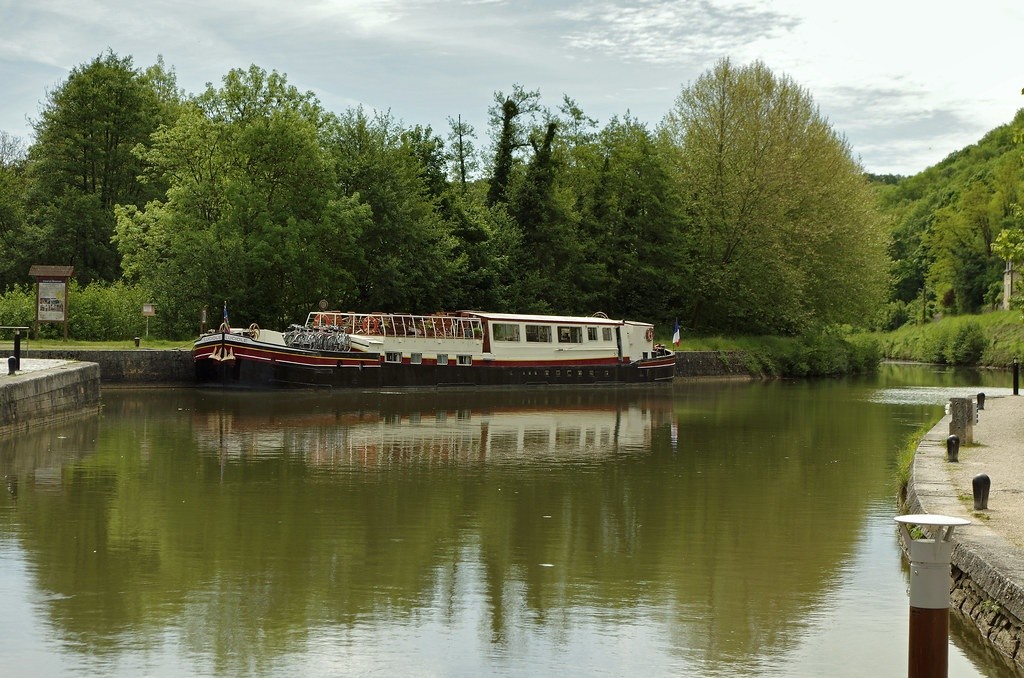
[672,317,680,347]
[218,301,230,334]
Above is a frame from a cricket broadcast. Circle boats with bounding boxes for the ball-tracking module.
[191,309,676,388]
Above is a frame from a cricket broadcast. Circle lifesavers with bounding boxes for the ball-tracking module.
[646,328,654,342]
[314,313,329,327]
[362,316,378,334]
